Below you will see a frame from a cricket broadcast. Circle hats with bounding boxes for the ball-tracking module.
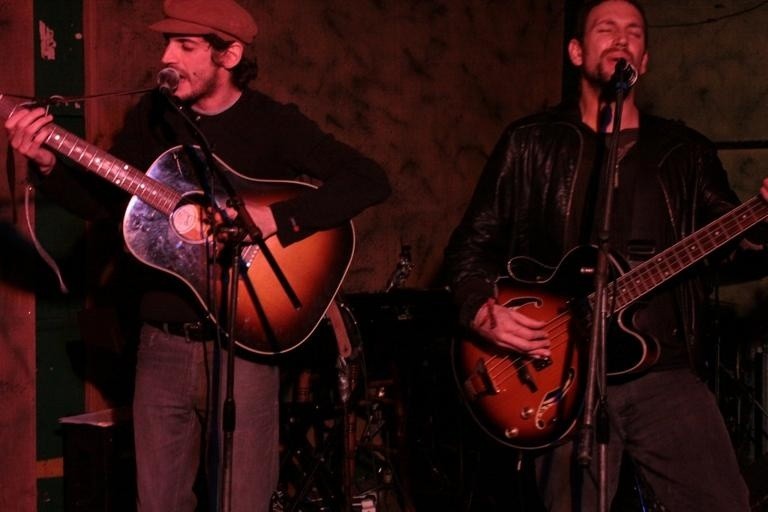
[147,0,259,45]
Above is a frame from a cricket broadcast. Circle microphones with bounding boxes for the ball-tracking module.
[612,58,639,88]
[154,67,180,96]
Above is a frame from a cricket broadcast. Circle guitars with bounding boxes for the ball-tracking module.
[449,192,766,450]
[0,96,356,357]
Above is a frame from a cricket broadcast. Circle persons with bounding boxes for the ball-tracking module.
[3,0,396,509]
[431,1,767,509]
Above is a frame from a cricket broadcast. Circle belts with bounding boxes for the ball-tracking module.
[146,320,215,343]
